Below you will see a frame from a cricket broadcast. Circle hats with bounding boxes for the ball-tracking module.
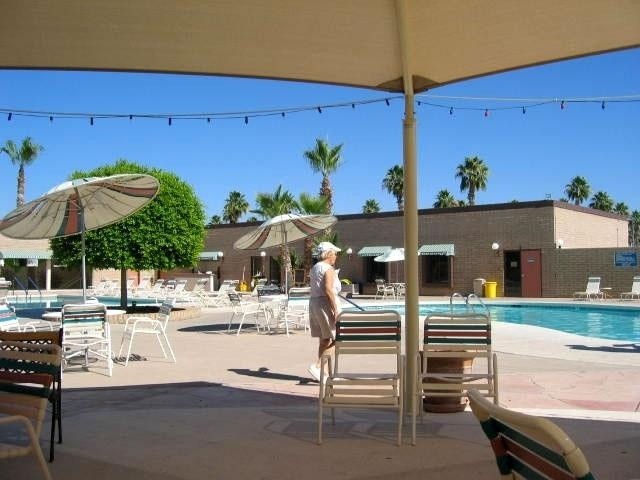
[317,242,341,252]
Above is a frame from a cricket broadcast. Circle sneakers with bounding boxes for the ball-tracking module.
[308,365,327,384]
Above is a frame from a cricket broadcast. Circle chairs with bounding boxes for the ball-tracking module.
[374,279,405,301]
[0,297,177,479]
[227,288,314,337]
[82,279,240,310]
[573,276,640,305]
[317,310,497,448]
[467,389,595,479]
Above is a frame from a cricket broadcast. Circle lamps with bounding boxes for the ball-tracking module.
[345,248,352,262]
[491,242,500,257]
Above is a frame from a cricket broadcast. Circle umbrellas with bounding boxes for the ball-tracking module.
[3,171,162,364]
[231,209,339,296]
[373,246,420,283]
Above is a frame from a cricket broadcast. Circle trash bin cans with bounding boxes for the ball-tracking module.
[483,282,497,298]
[240,280,248,292]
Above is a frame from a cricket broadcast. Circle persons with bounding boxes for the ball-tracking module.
[306,241,344,387]
